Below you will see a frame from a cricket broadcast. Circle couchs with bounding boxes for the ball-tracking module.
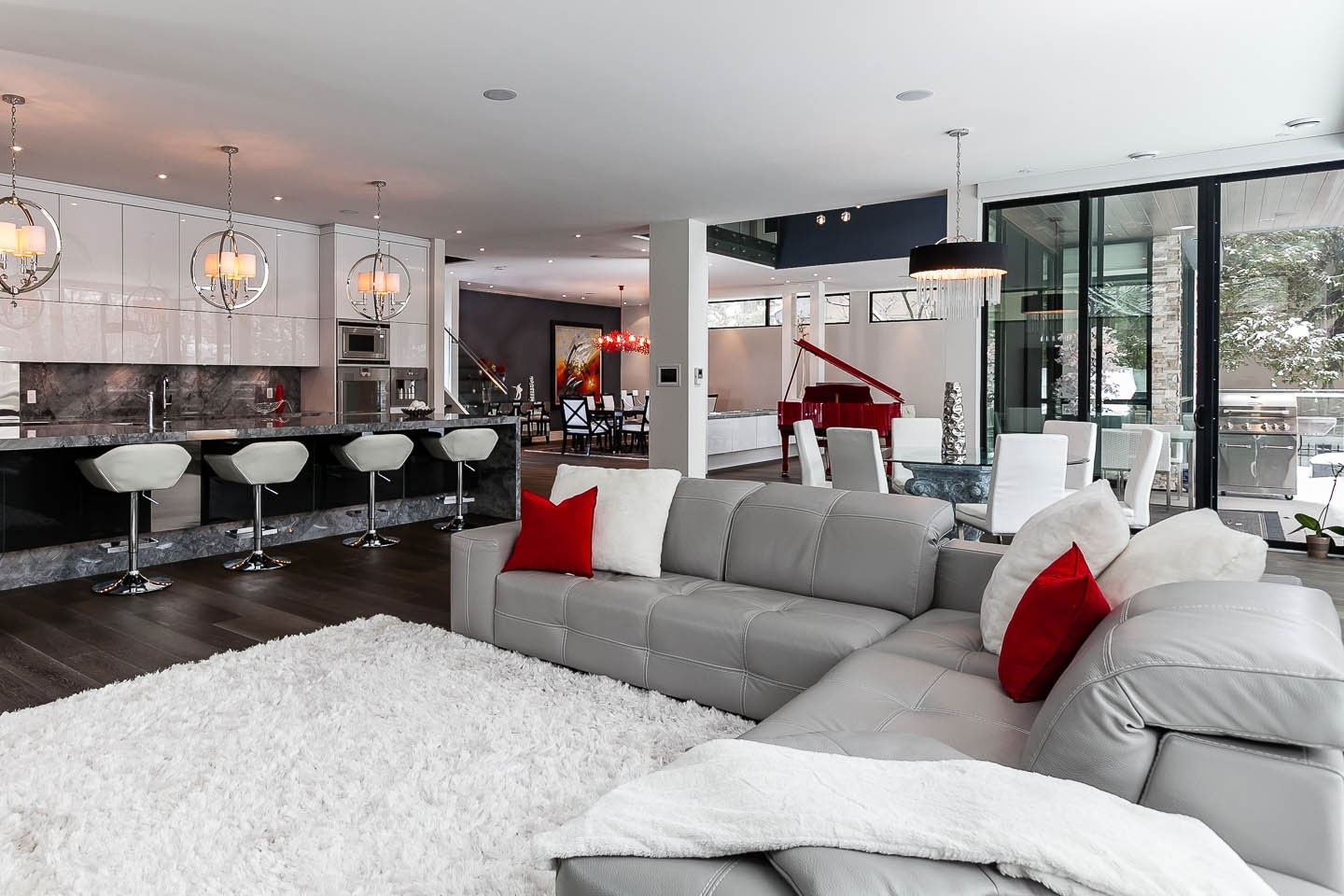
[555,539,1344,896]
[450,475,956,721]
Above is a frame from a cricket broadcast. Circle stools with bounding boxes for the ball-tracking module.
[74,427,498,595]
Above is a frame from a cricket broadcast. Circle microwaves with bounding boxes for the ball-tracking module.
[336,318,391,365]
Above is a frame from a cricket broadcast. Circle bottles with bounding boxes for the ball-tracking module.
[276,382,285,414]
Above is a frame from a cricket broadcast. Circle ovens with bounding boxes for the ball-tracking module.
[337,365,391,425]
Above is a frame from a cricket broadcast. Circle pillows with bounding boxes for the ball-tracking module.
[501,464,681,577]
[980,479,1269,703]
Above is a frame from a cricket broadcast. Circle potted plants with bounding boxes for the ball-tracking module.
[1288,463,1344,558]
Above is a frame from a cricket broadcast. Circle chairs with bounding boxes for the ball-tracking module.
[793,416,1184,544]
[559,392,718,455]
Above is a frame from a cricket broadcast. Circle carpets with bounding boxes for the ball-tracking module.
[0,614,758,896]
[1149,504,1286,542]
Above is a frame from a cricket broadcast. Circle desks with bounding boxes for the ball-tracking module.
[587,407,645,455]
[881,447,1090,541]
[1124,430,1197,510]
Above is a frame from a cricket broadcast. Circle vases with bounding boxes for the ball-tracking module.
[482,379,491,403]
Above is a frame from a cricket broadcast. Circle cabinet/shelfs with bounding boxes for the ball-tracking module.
[0,185,430,368]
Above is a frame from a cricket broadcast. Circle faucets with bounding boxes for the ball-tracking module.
[149,429,153,433]
[163,415,172,433]
[133,388,154,429]
[162,374,172,415]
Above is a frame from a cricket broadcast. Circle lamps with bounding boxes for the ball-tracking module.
[1020,218,1079,343]
[907,129,1008,321]
[592,285,649,354]
[190,146,270,321]
[0,94,62,308]
[346,181,411,332]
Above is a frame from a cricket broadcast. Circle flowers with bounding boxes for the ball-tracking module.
[476,359,506,382]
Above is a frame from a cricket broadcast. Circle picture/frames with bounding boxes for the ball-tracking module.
[550,320,603,411]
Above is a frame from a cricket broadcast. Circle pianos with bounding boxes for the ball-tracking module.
[777,338,905,480]
